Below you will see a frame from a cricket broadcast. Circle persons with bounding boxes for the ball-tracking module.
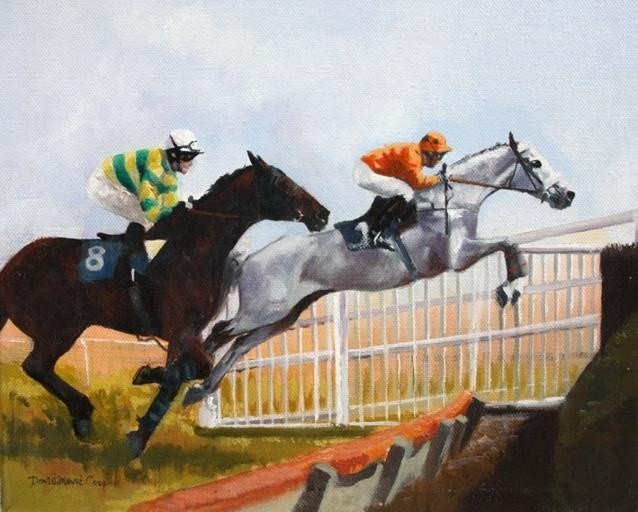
[333,132,453,255]
[89,130,205,291]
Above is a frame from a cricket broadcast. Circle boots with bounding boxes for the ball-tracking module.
[369,195,406,250]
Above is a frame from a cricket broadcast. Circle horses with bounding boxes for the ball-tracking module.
[0,150,331,456]
[181,130,575,408]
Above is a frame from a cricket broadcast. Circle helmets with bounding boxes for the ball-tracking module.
[163,127,204,155]
[419,130,451,154]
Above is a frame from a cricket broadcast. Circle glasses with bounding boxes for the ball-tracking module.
[175,152,196,161]
[428,154,442,160]
